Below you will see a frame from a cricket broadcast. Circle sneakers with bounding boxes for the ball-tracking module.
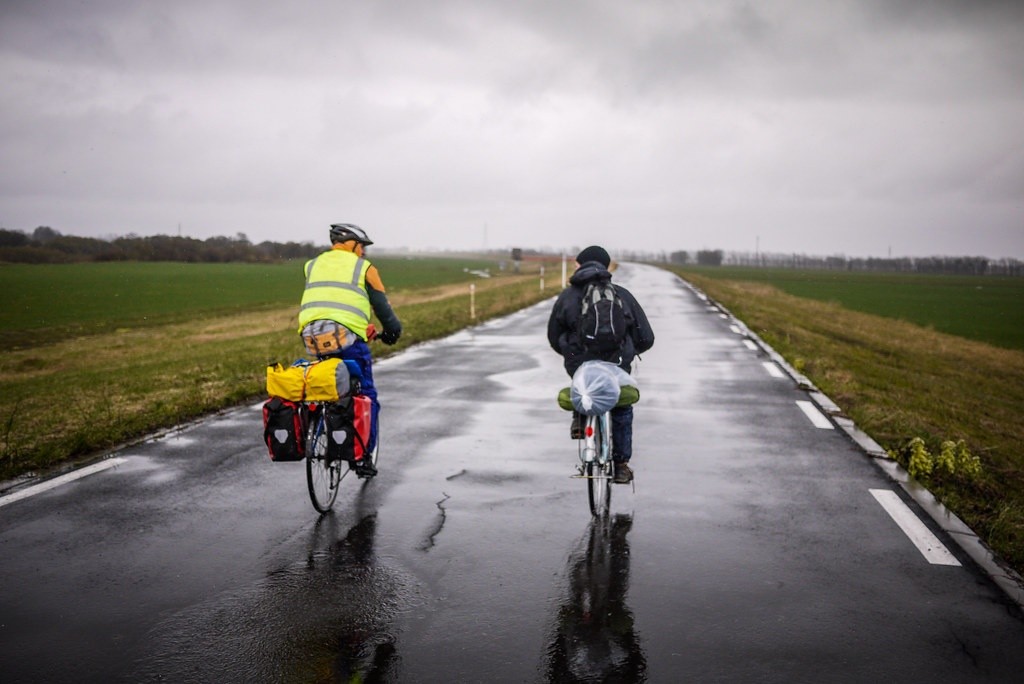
[613,464,634,484]
[348,456,377,476]
[571,418,585,439]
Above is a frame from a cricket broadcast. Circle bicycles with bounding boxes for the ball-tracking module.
[572,360,618,521]
[296,324,396,516]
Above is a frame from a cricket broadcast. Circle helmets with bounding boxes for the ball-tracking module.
[330,223,374,248]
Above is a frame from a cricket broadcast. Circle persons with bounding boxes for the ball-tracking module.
[548,245,654,480]
[297,223,403,479]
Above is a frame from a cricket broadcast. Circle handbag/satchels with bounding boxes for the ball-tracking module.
[572,359,638,415]
[558,387,641,411]
[261,398,309,462]
[322,393,371,462]
[300,319,358,356]
[265,356,351,401]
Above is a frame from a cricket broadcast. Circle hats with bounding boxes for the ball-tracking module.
[576,245,611,270]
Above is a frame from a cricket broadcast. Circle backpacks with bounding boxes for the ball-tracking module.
[574,278,628,351]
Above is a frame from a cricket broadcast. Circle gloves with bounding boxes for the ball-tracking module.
[380,330,400,345]
